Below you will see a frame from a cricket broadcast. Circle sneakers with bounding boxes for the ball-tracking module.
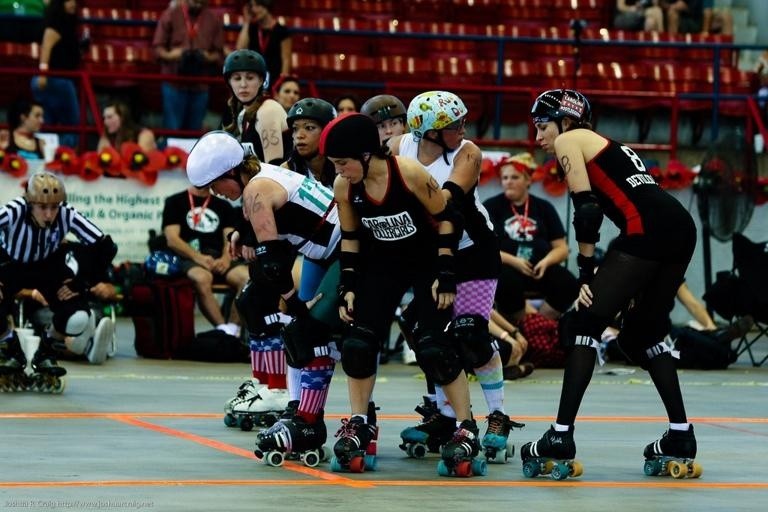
[86,317,114,365]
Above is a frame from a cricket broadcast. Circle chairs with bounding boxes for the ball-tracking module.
[1,1,767,144]
[700,229,768,369]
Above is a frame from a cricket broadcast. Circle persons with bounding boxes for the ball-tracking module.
[153,1,290,131]
[161,50,716,480]
[1,0,157,159]
[0,173,123,391]
[614,0,731,39]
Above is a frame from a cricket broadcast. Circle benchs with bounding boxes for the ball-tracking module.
[129,277,245,359]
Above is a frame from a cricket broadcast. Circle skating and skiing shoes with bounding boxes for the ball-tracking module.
[403,395,525,477]
[520,426,584,480]
[224,377,332,467]
[1,328,67,395]
[643,423,703,478]
[330,402,380,474]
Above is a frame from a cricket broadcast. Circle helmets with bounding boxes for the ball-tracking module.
[531,89,593,122]
[286,91,468,157]
[222,48,268,76]
[186,129,245,187]
[25,172,66,205]
